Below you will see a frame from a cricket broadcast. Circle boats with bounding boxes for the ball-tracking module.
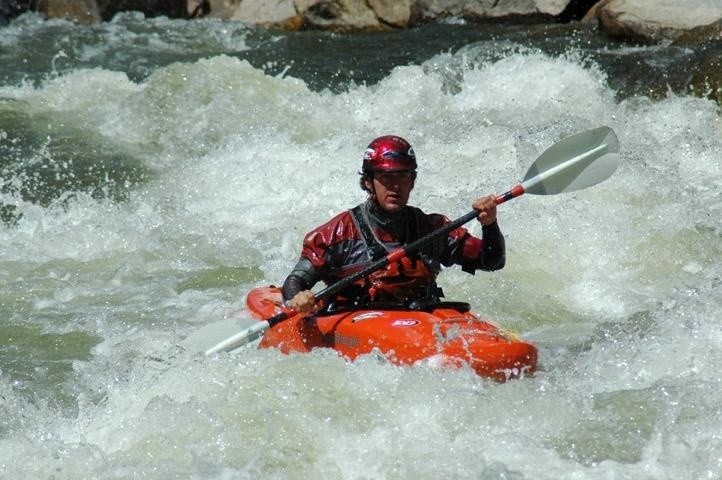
[245,284,538,385]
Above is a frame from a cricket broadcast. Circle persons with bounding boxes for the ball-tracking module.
[283,134,506,314]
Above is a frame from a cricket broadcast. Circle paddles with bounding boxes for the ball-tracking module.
[203,126,620,360]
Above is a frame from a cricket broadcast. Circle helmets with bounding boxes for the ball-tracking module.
[363,136,417,171]
